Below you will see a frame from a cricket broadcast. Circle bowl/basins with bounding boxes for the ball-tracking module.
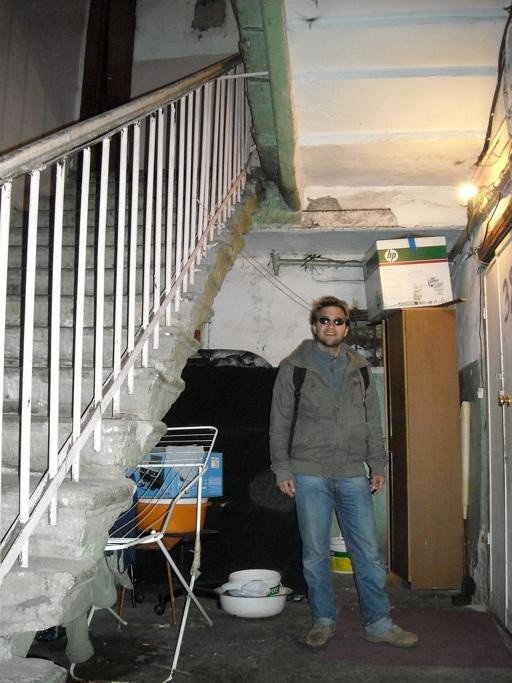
[330,537,355,575]
[134,501,214,534]
[212,585,294,620]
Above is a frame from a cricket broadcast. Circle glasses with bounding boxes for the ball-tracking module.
[319,317,345,325]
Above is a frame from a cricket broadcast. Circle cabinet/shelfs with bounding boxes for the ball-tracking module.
[381,308,462,597]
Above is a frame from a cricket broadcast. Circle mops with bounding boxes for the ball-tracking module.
[450,519,475,607]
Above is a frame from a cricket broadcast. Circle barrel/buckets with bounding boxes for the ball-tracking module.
[329,531,355,575]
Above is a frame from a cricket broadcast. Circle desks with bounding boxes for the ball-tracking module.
[115,536,186,633]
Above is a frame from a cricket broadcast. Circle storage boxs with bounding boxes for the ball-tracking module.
[361,236,453,322]
[125,452,224,499]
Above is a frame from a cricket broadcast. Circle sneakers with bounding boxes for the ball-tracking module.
[304,622,337,648]
[363,621,419,647]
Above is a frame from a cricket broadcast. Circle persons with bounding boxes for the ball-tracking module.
[266,294,420,649]
